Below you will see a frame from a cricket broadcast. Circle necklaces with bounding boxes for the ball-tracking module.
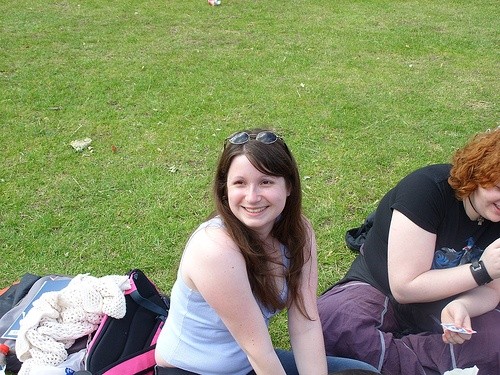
[466,196,486,225]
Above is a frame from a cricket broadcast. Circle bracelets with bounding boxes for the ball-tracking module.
[468,258,494,286]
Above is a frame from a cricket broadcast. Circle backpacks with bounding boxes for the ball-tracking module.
[81,268,170,375]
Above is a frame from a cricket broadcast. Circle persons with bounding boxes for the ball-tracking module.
[314,129,500,375]
[154,127,382,375]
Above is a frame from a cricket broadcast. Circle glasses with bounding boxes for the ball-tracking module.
[224,131,292,163]
